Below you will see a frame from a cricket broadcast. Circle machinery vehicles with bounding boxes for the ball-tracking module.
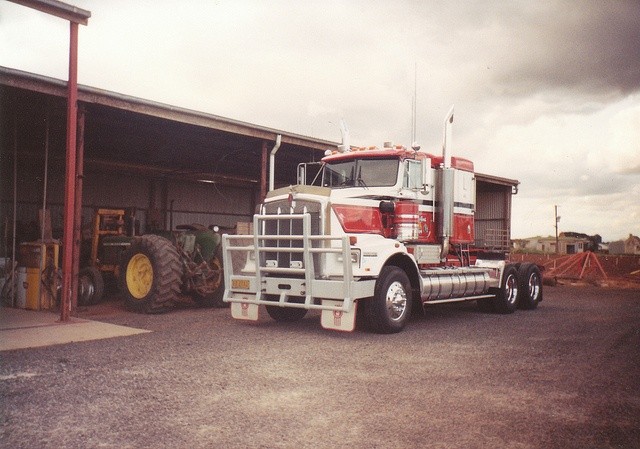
[78,199,231,314]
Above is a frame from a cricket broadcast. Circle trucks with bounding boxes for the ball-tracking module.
[221,105,543,334]
